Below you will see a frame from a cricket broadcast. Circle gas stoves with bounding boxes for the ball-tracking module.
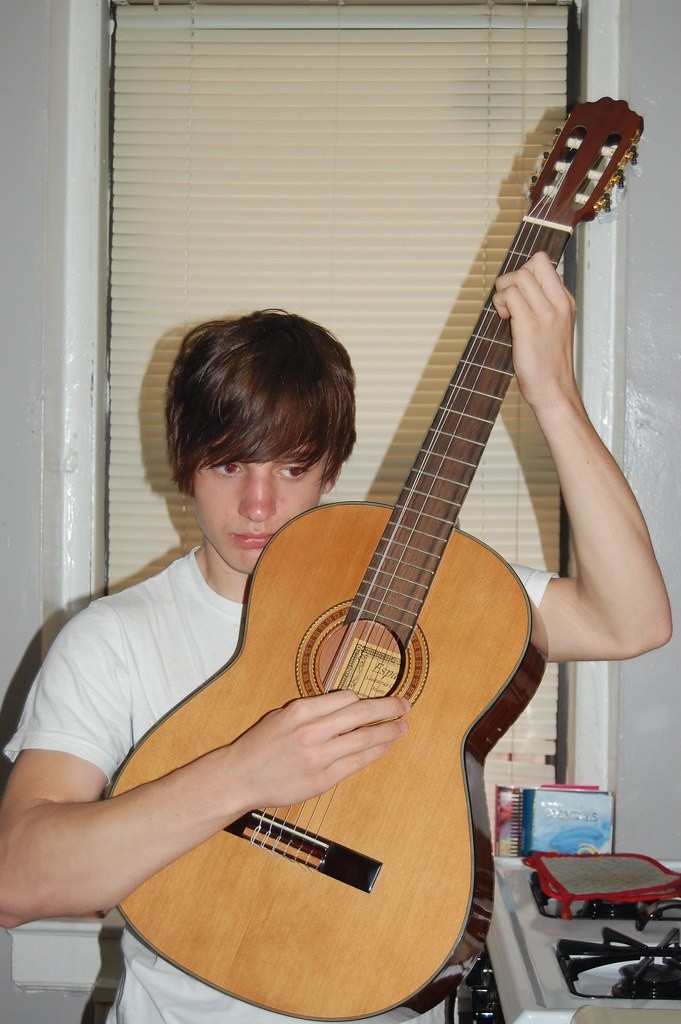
[469,857,681,1024]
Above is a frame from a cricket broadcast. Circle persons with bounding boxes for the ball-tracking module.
[0,252,673,1024]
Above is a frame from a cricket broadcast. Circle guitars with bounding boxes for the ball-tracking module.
[106,93,648,1024]
